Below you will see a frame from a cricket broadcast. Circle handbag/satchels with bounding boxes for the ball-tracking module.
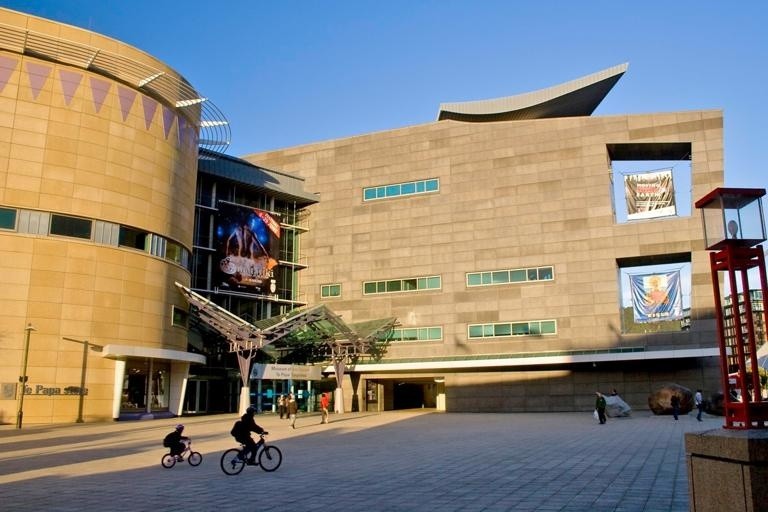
[231,422,242,435]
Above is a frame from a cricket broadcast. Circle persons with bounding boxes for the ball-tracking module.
[695,389,703,421]
[610,388,618,396]
[276,392,298,429]
[595,392,607,424]
[231,406,263,465]
[163,424,190,462]
[671,392,681,420]
[320,393,329,424]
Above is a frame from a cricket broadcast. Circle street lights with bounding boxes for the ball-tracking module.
[692,181,766,428]
[14,323,39,431]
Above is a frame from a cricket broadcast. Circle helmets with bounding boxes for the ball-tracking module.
[247,408,256,413]
[176,425,184,431]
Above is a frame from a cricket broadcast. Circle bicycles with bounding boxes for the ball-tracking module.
[160,438,205,470]
[218,431,286,477]
